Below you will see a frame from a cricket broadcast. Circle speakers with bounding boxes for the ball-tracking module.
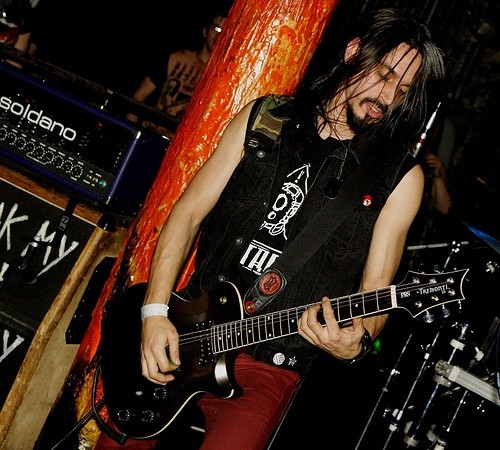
[0,177,96,413]
[0,59,172,229]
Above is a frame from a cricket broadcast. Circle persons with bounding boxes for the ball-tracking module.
[416,93,488,250]
[119,10,232,163]
[90,23,430,449]
[0,0,41,71]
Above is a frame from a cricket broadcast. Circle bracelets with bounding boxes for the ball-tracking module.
[141,302,171,318]
[349,324,373,365]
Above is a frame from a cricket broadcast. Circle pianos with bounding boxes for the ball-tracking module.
[98,269,470,439]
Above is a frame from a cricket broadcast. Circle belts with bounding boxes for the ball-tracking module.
[242,338,311,371]
